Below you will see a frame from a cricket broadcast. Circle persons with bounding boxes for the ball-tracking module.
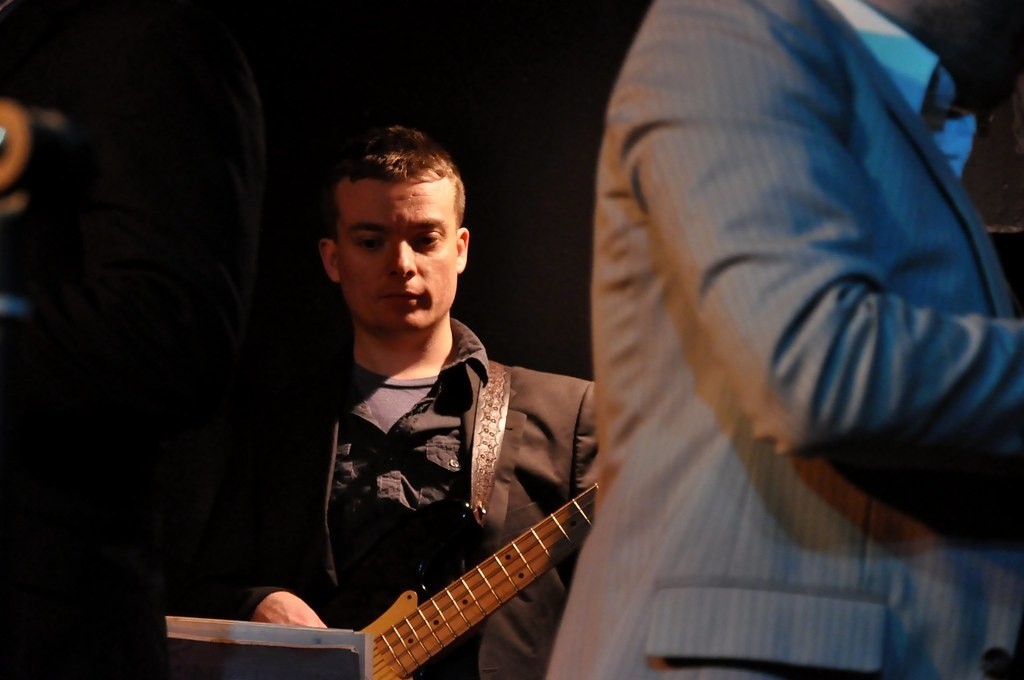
[543,0,1024,680]
[187,125,596,680]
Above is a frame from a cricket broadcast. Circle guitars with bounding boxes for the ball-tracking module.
[356,482,598,679]
